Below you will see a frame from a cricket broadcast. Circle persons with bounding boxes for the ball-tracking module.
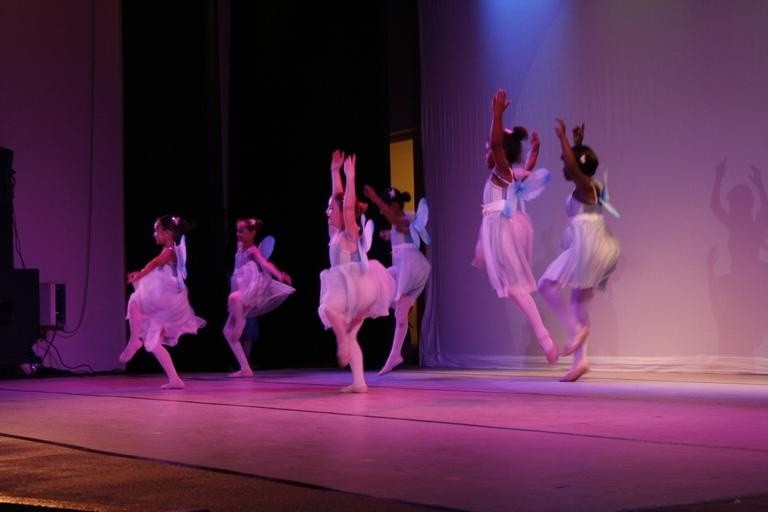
[537,117,622,382]
[362,183,432,376]
[317,151,394,392]
[223,219,294,378]
[471,88,559,364]
[120,215,207,389]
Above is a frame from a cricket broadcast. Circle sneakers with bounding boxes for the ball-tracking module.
[561,322,591,358]
[339,382,370,394]
[538,334,559,362]
[228,369,254,379]
[337,334,355,368]
[231,318,248,341]
[395,295,415,327]
[159,379,187,390]
[560,362,591,381]
[376,355,406,377]
[118,339,144,364]
